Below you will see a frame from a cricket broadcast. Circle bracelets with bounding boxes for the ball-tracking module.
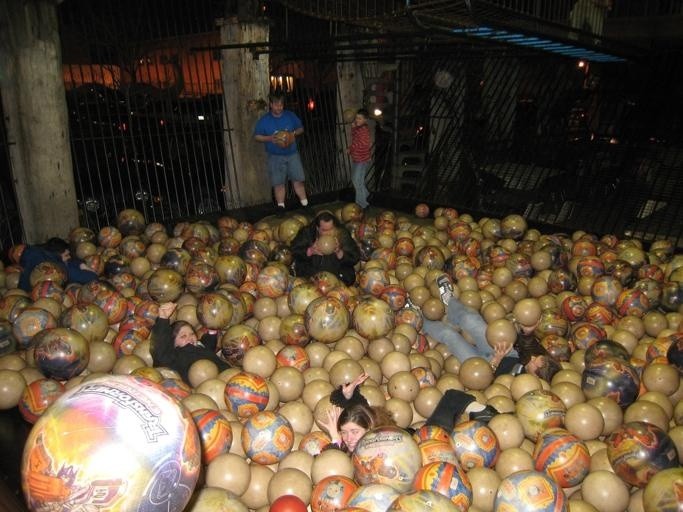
[294,131,297,137]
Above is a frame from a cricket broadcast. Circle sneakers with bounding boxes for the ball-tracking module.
[438,274,453,305]
[303,204,313,214]
[276,205,286,218]
[469,404,498,421]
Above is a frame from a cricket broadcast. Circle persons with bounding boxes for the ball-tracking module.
[289,213,360,288]
[253,94,312,207]
[17,238,99,292]
[150,301,233,387]
[403,273,563,387]
[317,374,500,457]
[344,109,371,208]
